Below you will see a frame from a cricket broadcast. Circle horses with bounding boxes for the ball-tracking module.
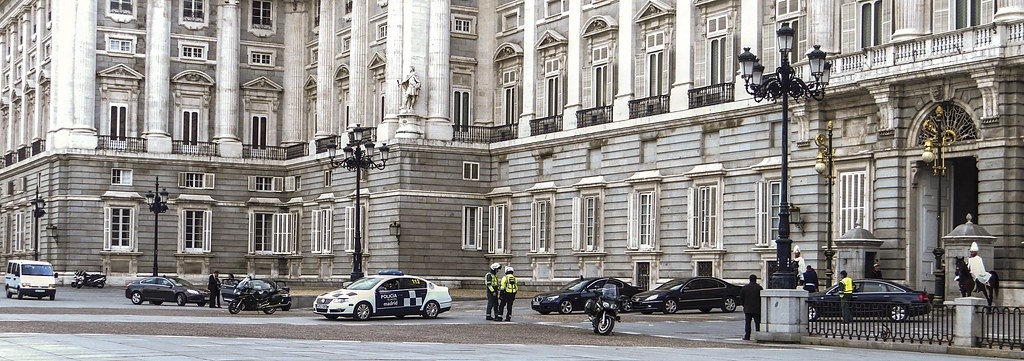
[955,255,999,315]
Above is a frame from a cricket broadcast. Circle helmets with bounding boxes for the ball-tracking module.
[491,263,501,271]
[505,266,514,273]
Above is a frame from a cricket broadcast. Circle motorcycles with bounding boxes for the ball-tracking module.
[228,273,282,316]
[71,268,107,288]
[583,283,626,335]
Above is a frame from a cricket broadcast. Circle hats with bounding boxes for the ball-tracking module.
[970,242,979,252]
[874,264,879,267]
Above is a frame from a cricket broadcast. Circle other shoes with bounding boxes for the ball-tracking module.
[742,338,749,340]
[505,318,510,321]
[210,306,217,308]
[486,316,493,319]
[494,316,503,321]
[218,306,222,308]
[842,321,848,324]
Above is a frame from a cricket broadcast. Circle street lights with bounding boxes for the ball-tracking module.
[145,175,169,276]
[328,124,390,286]
[921,106,956,307]
[738,23,833,290]
[815,121,836,291]
[31,185,46,261]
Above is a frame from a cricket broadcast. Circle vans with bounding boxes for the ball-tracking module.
[5,260,58,300]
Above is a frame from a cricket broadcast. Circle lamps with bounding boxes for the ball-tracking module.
[45,223,60,244]
[389,221,402,246]
[789,203,805,237]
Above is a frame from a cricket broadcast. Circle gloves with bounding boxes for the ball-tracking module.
[839,294,844,298]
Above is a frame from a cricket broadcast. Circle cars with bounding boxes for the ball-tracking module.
[221,276,292,311]
[806,278,932,322]
[630,276,741,314]
[532,274,642,315]
[126,278,211,307]
[313,274,453,321]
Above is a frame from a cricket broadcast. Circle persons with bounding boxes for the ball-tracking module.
[870,264,882,279]
[398,65,421,110]
[803,265,819,292]
[968,241,991,291]
[739,274,763,341]
[838,271,857,326]
[484,263,504,320]
[793,245,806,286]
[494,266,519,322]
[207,271,222,308]
